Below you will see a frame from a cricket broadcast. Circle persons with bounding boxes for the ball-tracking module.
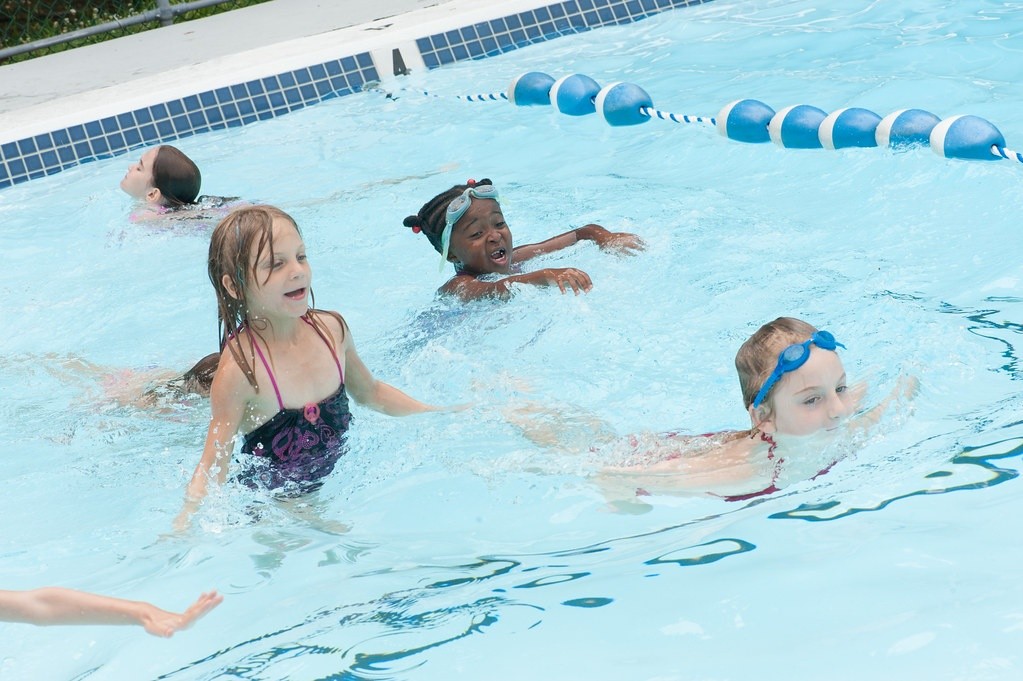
[120,146,202,212]
[403,178,649,303]
[736,317,853,445]
[0,587,224,638]
[183,204,439,496]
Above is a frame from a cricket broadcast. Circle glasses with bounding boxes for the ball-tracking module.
[753,330,848,409]
[439,184,509,273]
